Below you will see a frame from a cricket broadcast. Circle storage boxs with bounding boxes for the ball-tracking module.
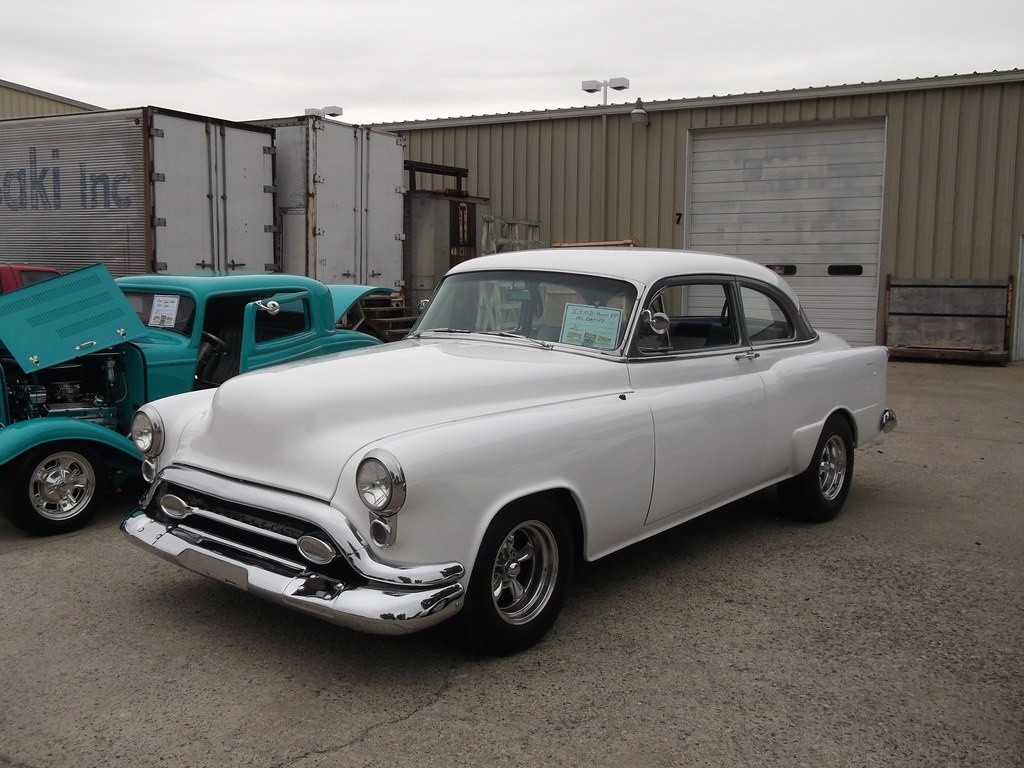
[235,115,408,319]
[2,107,276,281]
[402,190,494,319]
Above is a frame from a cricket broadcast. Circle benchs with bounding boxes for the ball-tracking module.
[638,317,790,347]
[196,320,299,385]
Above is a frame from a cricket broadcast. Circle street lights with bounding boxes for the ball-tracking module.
[304,106,343,122]
[581,77,630,107]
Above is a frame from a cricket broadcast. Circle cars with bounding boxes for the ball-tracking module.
[119,245,898,657]
[0,260,403,537]
[0,265,64,298]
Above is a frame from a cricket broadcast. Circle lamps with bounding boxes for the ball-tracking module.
[630,97,649,128]
